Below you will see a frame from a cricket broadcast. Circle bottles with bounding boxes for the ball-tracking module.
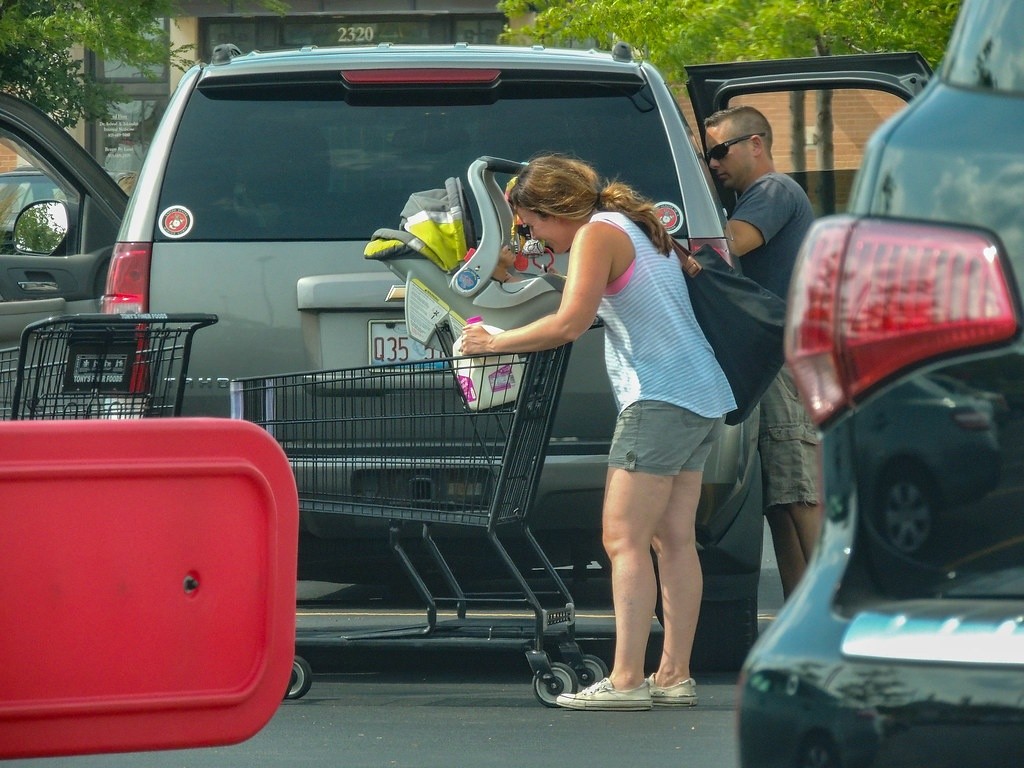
[451,316,524,412]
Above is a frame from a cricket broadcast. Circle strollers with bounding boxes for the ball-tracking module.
[362,155,568,358]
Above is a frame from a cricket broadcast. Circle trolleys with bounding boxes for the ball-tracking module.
[227,316,610,710]
[0,312,221,422]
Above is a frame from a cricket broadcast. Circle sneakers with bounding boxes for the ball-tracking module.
[557,677,652,710]
[648,673,698,706]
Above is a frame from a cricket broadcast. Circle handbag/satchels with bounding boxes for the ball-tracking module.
[670,236,788,425]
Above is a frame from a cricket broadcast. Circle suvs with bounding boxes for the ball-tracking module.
[96,39,937,676]
[731,0,1024,768]
[0,89,130,421]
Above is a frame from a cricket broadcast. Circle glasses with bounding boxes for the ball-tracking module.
[705,132,766,165]
[518,217,542,241]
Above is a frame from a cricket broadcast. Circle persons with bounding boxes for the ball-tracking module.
[703,105,828,600]
[490,245,521,283]
[457,153,740,709]
